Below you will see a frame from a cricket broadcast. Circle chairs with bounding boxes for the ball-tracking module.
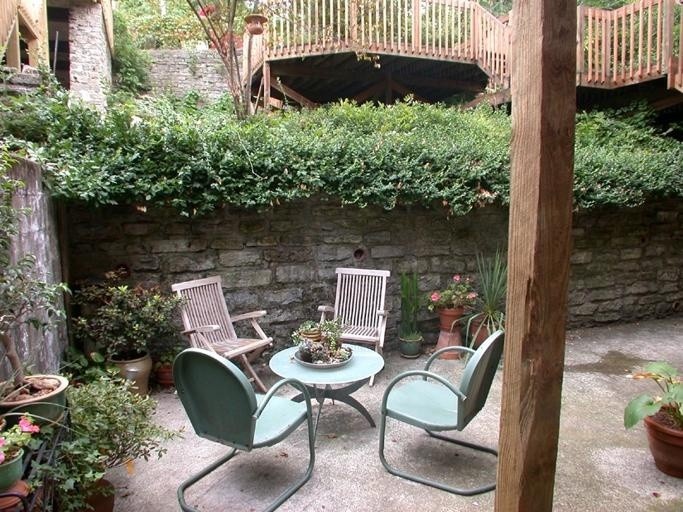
[315,262,393,388]
[372,328,508,499]
[165,271,273,396]
[166,345,319,511]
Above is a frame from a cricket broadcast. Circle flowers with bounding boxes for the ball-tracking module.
[423,275,479,315]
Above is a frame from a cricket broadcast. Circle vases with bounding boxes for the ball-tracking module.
[427,304,467,362]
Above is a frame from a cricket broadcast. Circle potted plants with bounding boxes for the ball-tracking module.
[0,413,41,512]
[1,140,70,426]
[42,375,186,512]
[443,244,506,369]
[613,358,682,479]
[75,268,190,398]
[243,7,270,36]
[394,262,427,360]
[289,315,353,367]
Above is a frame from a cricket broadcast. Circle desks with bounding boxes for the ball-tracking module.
[265,341,386,430]
